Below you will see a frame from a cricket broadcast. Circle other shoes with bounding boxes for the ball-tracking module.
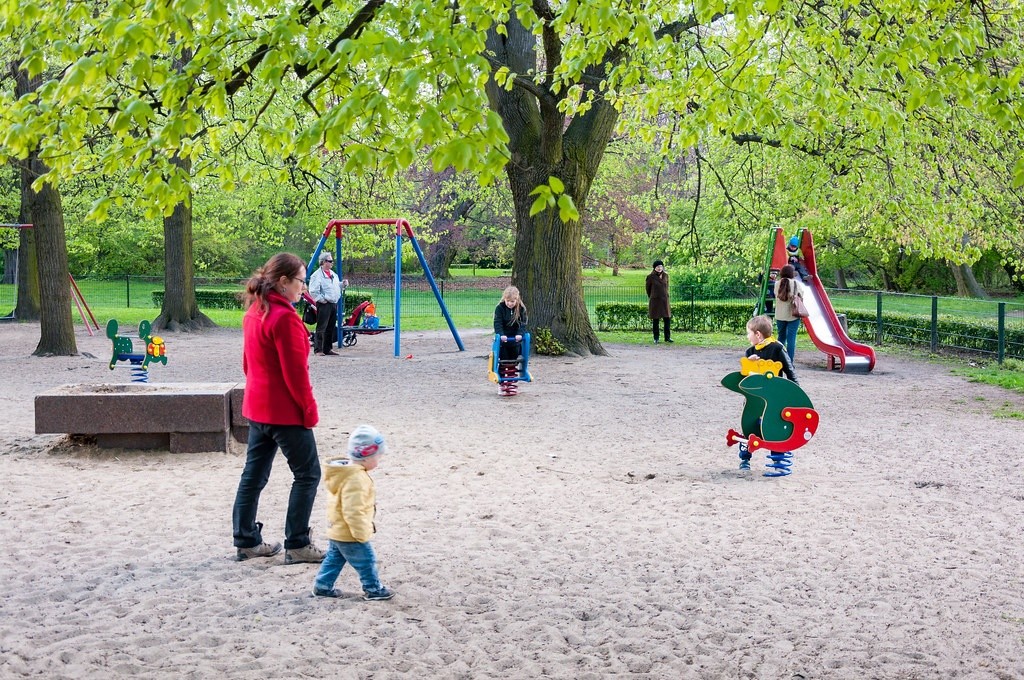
[664,339,674,342]
[324,351,339,355]
[653,340,659,344]
[803,275,813,280]
[498,385,516,394]
[315,352,325,355]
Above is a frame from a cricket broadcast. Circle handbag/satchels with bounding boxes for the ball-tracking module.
[790,281,809,318]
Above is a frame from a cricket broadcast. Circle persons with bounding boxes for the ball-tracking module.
[311,425,396,601]
[739,316,800,470]
[774,264,800,369]
[645,260,674,344]
[786,237,813,282]
[360,304,379,335]
[758,269,778,321]
[232,253,328,565]
[309,252,349,356]
[494,286,528,386]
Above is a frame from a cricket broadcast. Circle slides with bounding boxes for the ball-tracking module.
[769,228,876,374]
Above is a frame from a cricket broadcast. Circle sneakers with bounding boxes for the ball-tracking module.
[285,527,328,565]
[363,586,396,600]
[236,522,281,561]
[312,587,349,598]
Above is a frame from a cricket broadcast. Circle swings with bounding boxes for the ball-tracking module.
[341,224,396,335]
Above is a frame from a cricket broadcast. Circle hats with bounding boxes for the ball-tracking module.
[349,425,385,460]
[789,236,800,247]
[364,304,375,314]
[653,261,663,268]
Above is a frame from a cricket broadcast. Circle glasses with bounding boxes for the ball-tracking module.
[294,277,306,285]
[326,260,332,263]
